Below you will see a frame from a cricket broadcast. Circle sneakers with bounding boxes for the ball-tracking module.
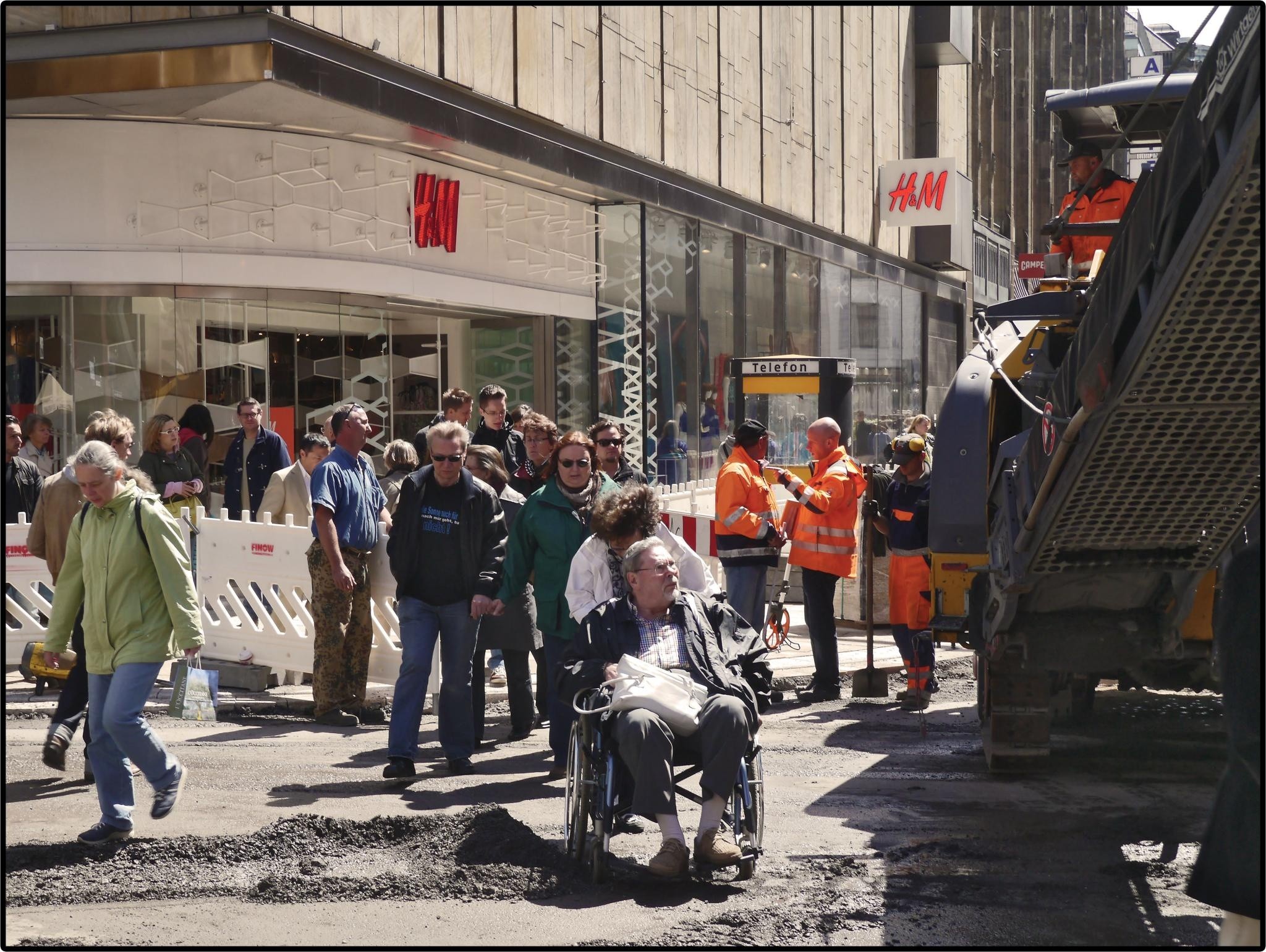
[150,764,187,819]
[490,664,508,684]
[693,830,742,866]
[649,836,691,879]
[77,821,134,845]
[614,811,645,833]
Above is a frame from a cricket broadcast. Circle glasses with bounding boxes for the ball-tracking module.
[345,402,364,419]
[557,456,589,468]
[596,438,621,447]
[431,450,463,462]
[608,540,630,553]
[128,441,135,448]
[160,426,181,435]
[636,560,680,576]
[239,412,260,420]
[482,407,509,417]
[521,437,548,446]
[466,464,486,471]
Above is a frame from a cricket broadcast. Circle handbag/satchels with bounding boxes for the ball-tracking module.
[572,655,709,735]
[164,494,208,531]
[167,652,219,723]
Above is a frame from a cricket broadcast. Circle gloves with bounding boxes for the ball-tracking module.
[861,499,881,523]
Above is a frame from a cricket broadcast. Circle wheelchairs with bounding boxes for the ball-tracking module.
[561,647,771,881]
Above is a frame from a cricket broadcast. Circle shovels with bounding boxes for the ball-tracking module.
[852,467,888,698]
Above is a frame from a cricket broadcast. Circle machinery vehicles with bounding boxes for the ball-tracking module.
[914,1,1266,780]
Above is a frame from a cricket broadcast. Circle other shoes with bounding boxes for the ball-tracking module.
[535,716,550,729]
[383,755,416,779]
[507,714,536,740]
[316,708,359,726]
[895,684,930,710]
[85,760,141,780]
[348,705,388,722]
[448,758,477,775]
[794,675,840,703]
[547,763,573,779]
[41,736,67,771]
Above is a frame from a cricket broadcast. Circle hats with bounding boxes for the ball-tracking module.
[734,419,776,445]
[1056,143,1103,167]
[890,432,925,466]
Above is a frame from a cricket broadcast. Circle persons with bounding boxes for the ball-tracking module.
[299,400,393,727]
[713,417,788,634]
[384,419,508,779]
[774,417,868,703]
[5,384,945,781]
[544,536,772,878]
[1045,142,1136,263]
[220,397,293,524]
[46,440,205,846]
